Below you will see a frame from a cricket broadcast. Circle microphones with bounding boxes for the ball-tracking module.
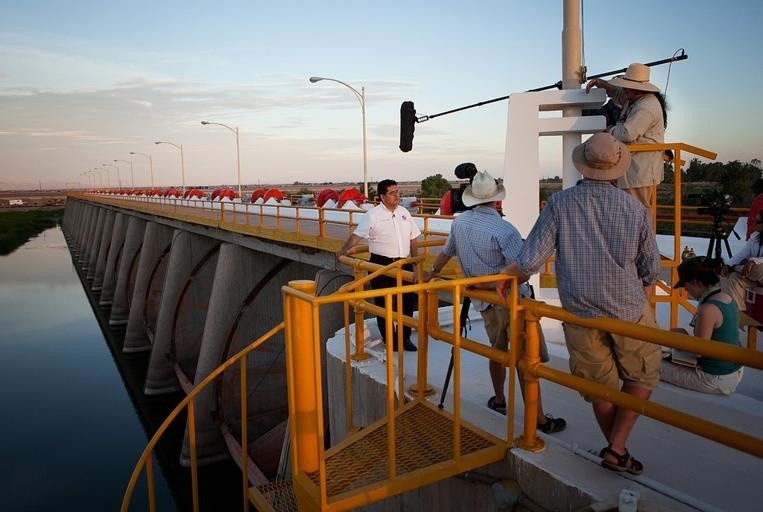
[399,102,415,152]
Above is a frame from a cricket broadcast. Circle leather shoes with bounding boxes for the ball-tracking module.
[386,334,417,351]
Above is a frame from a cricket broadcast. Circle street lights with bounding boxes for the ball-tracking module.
[201,120,241,198]
[128,152,154,191]
[155,142,186,193]
[77,159,134,192]
[309,75,370,199]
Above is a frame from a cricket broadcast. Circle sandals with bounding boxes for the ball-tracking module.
[536,417,567,434]
[488,394,507,413]
[602,443,644,476]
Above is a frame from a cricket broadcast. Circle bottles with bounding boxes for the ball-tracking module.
[681,245,697,260]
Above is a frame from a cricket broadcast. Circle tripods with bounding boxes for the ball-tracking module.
[707,227,732,259]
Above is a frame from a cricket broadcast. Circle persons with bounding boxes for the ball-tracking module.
[585,62,668,214]
[719,207,763,312]
[496,130,665,476]
[419,169,568,436]
[332,178,422,353]
[654,254,747,397]
[555,73,623,128]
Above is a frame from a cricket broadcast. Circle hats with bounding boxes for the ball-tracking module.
[607,62,661,93]
[573,132,632,179]
[672,255,712,287]
[462,170,505,207]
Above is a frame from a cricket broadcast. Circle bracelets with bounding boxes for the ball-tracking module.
[431,264,440,273]
